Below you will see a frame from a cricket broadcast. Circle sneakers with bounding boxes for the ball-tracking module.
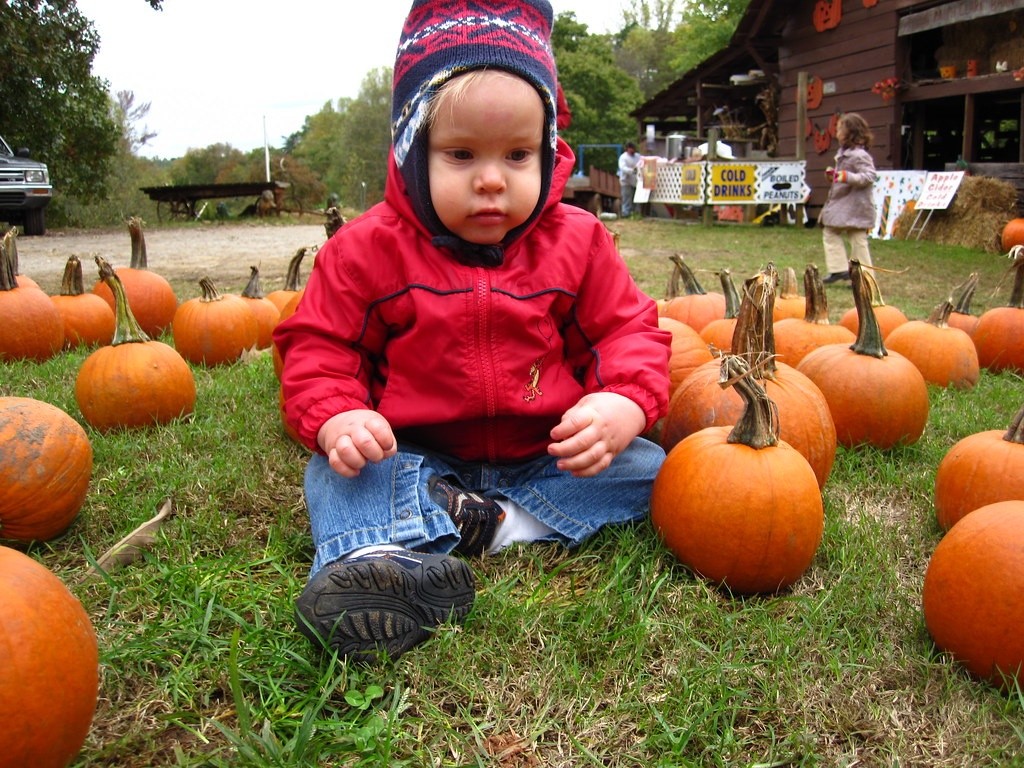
[294,550,476,669]
[421,474,508,559]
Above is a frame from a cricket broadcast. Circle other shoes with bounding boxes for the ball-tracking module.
[820,272,851,283]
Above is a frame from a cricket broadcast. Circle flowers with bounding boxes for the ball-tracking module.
[870,75,909,103]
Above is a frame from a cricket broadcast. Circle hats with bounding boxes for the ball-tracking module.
[389,0,560,256]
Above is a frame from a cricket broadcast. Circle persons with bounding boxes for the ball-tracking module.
[619,142,642,218]
[821,116,878,287]
[277,0,676,662]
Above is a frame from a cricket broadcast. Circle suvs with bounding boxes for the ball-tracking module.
[0,135,52,232]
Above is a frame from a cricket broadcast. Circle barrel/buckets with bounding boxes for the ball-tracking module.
[668,135,685,159]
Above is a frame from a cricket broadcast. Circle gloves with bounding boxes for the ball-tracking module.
[832,170,850,184]
[824,165,835,181]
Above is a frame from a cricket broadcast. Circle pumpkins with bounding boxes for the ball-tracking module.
[652,218,1024,699]
[0,218,309,768]
